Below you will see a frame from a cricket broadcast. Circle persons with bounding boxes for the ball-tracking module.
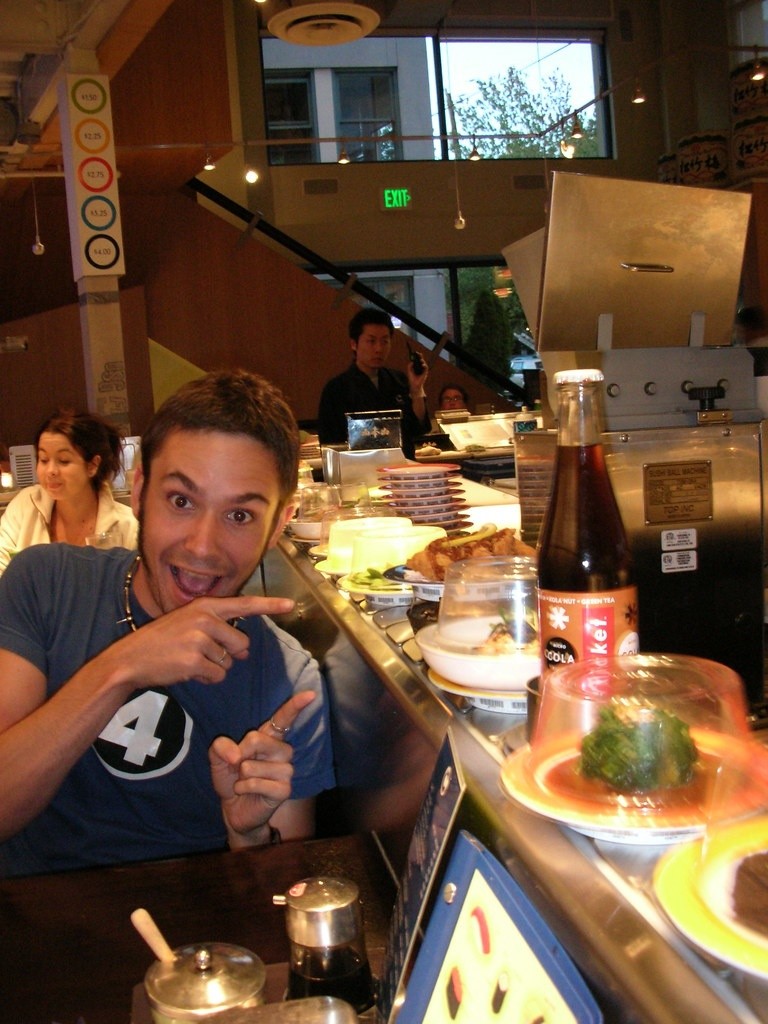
[0,367,338,883]
[0,403,139,580]
[437,383,470,411]
[317,306,433,469]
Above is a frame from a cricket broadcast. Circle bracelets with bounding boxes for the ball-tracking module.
[408,393,427,400]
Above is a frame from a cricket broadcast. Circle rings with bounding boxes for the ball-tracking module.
[270,716,291,734]
[216,648,227,665]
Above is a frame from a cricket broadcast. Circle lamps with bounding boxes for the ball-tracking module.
[244,163,261,184]
[337,141,351,164]
[468,135,481,162]
[203,148,216,171]
[571,111,584,138]
[27,140,47,257]
[631,74,651,104]
[449,139,468,231]
[748,49,767,86]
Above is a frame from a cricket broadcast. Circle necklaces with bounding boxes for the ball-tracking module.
[123,552,248,635]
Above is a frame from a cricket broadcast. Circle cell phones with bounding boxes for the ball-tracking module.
[405,342,425,376]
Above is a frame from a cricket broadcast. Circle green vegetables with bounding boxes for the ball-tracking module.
[578,705,701,794]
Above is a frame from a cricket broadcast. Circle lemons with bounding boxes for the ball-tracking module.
[440,522,498,547]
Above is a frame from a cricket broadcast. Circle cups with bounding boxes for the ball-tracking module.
[525,676,544,744]
[85,532,123,548]
[144,942,268,1024]
[124,467,136,493]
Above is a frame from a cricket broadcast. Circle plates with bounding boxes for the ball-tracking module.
[652,813,768,979]
[426,668,529,714]
[502,728,768,845]
[290,537,321,546]
[308,545,328,562]
[112,489,130,496]
[383,564,537,600]
[337,574,414,604]
[314,559,350,581]
[377,463,474,536]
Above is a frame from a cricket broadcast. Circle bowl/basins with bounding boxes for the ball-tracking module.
[411,434,449,452]
[416,616,541,690]
[289,519,320,539]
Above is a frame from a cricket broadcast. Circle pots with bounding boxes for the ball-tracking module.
[407,597,443,634]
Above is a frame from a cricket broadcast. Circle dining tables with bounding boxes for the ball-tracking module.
[0,832,392,1024]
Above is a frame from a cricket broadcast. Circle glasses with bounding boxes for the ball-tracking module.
[441,395,462,403]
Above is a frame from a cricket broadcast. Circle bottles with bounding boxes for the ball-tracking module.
[273,876,375,1015]
[536,369,639,699]
[517,406,533,421]
